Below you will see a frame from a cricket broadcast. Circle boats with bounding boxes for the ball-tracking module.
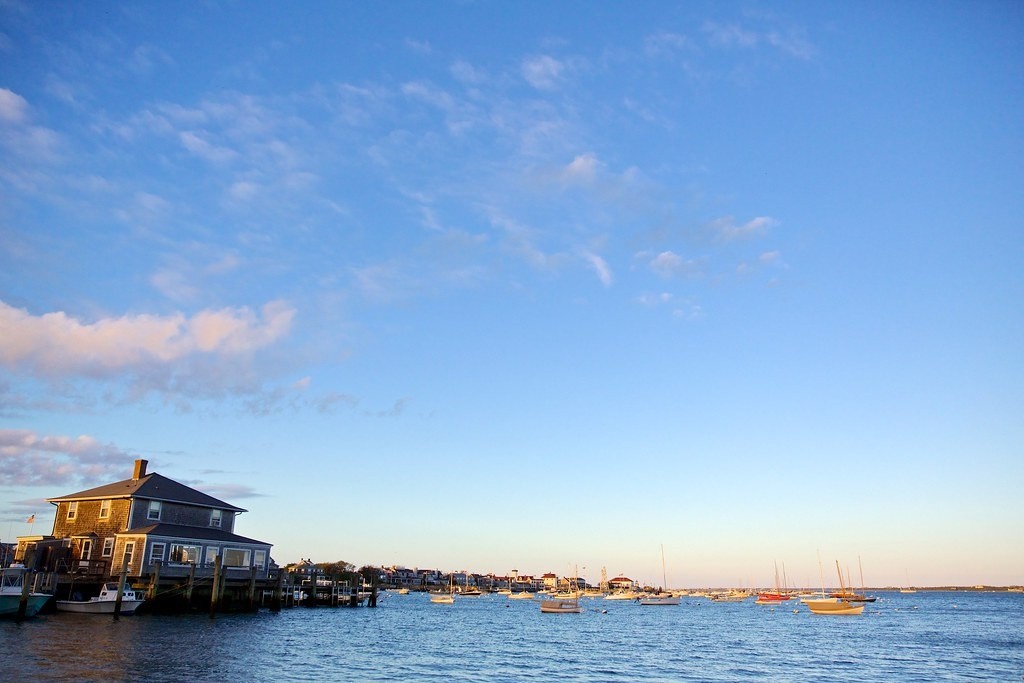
[542,571,604,599]
[54,581,147,615]
[584,588,602,598]
[608,588,699,600]
[0,562,53,619]
[509,590,535,599]
[639,599,679,606]
[459,583,479,595]
[498,587,511,595]
[431,595,455,605]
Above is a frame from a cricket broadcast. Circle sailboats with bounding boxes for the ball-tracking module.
[540,564,584,613]
[801,560,865,616]
[830,558,857,597]
[756,562,795,604]
[841,555,876,602]
